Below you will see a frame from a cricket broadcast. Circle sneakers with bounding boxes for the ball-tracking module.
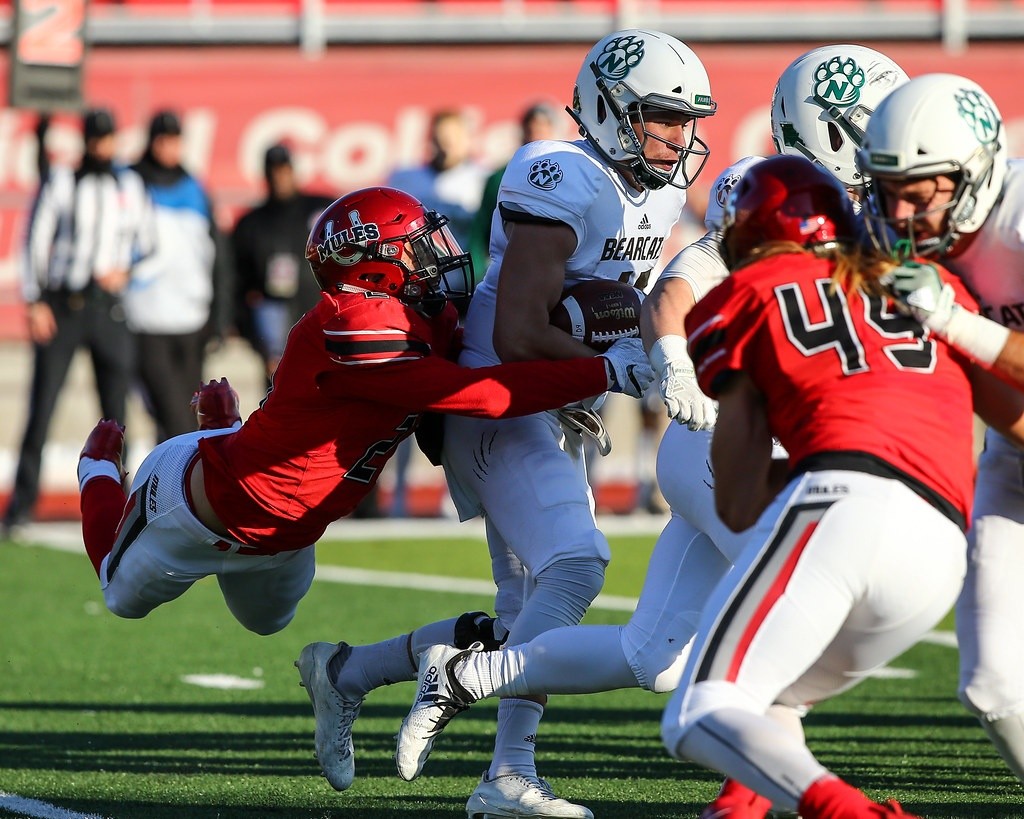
[188,375,243,425]
[464,771,592,817]
[394,641,480,782]
[295,642,365,793]
[75,416,128,472]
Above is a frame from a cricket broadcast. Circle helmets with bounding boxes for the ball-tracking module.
[713,155,856,256]
[571,31,716,190]
[861,69,1010,236]
[769,42,911,189]
[301,185,476,318]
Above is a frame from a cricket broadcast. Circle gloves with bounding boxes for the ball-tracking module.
[604,337,655,397]
[894,259,1011,370]
[651,336,717,431]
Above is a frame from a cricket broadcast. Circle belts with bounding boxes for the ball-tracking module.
[215,540,283,556]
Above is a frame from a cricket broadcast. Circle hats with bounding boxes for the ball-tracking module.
[149,112,183,140]
[262,143,291,176]
[83,106,114,143]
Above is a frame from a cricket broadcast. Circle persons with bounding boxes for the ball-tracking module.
[1,100,670,537]
[79,29,719,819]
[395,44,1024,819]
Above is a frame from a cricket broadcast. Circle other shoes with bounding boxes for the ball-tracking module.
[631,482,670,515]
[7,492,34,523]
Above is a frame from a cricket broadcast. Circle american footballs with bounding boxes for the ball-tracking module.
[548,279,647,353]
